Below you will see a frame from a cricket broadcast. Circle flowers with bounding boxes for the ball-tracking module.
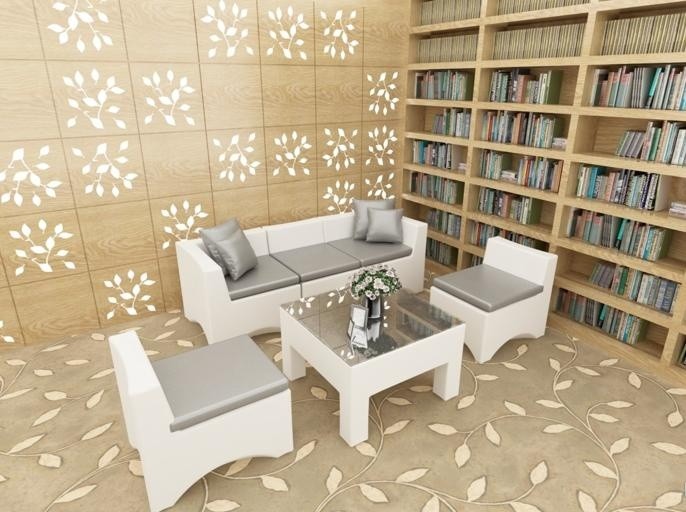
[345,262,404,302]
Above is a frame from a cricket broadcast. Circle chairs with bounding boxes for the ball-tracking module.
[428,235,558,369]
[108,331,297,512]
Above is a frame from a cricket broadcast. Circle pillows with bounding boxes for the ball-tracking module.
[198,214,246,280]
[212,229,260,287]
[351,195,397,241]
[364,205,405,244]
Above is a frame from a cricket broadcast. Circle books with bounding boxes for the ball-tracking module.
[415,69,472,138]
[591,66,685,164]
[493,0,590,60]
[568,165,686,260]
[425,209,461,264]
[600,11,686,54]
[408,138,467,203]
[478,149,564,222]
[416,0,481,62]
[471,221,544,266]
[481,70,567,150]
[560,261,680,344]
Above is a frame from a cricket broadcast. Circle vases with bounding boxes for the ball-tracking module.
[361,293,385,320]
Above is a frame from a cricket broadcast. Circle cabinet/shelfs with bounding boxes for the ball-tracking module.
[395,0,685,390]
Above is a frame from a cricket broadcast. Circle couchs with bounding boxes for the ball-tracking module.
[169,203,434,347]
